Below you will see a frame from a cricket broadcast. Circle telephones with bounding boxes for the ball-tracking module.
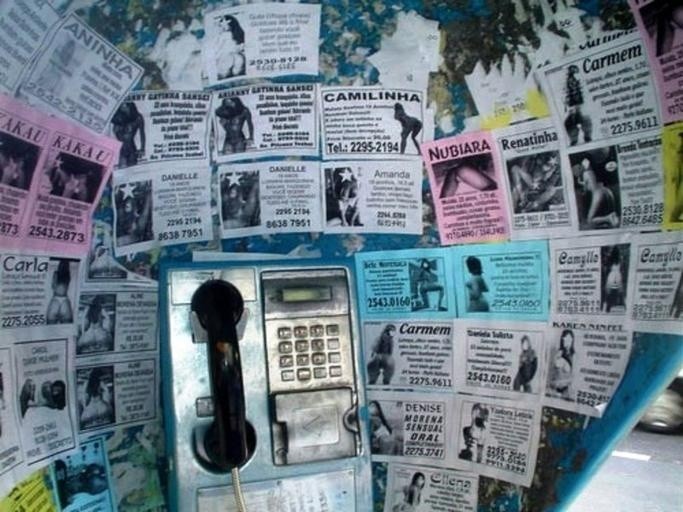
[191,280,249,470]
